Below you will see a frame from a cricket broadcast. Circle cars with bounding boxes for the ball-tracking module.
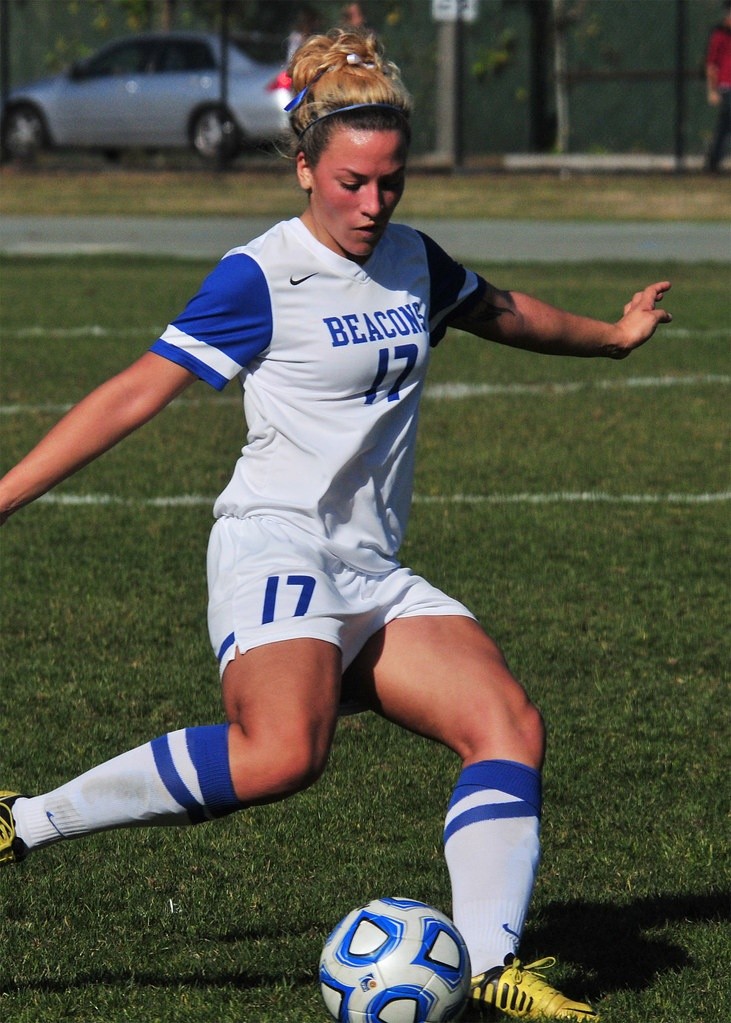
[6,27,304,163]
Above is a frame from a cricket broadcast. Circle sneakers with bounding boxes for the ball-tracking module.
[466,953,600,1023]
[0,789,29,867]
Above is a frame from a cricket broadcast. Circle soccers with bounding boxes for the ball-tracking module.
[319,896,472,1023]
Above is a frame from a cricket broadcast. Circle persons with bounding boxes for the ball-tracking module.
[0,27,671,1021]
[698,12,731,174]
